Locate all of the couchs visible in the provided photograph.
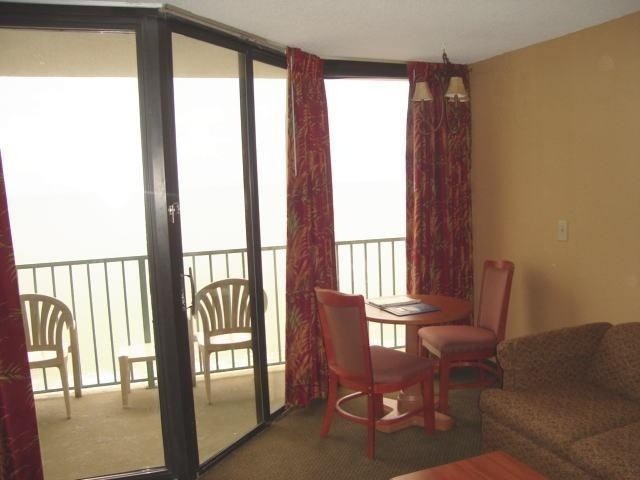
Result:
[476,321,640,480]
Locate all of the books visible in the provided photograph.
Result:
[364,295,441,317]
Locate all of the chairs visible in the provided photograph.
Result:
[118,277,269,410]
[15,293,81,421]
[313,285,435,461]
[419,260,514,414]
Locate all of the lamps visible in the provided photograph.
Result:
[409,48,472,136]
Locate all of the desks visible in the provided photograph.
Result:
[359,294,473,432]
